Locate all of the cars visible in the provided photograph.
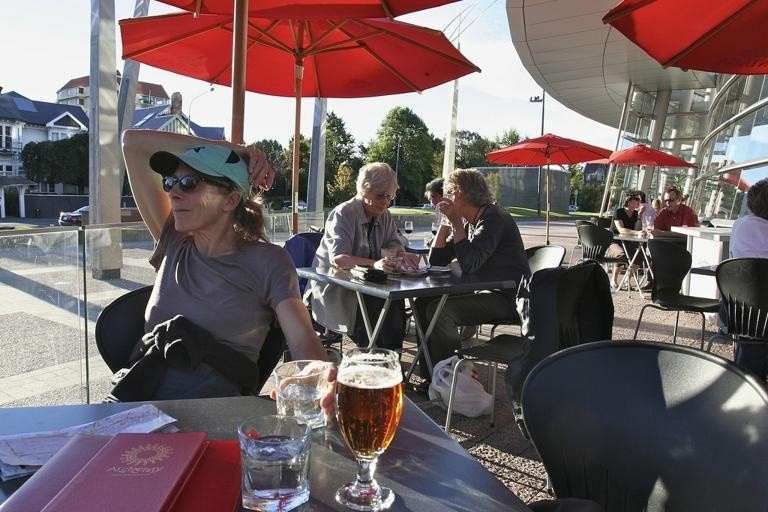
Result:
[58,205,90,226]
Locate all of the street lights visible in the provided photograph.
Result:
[187,87,215,136]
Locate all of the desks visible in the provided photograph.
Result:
[671,225,732,300]
[406,239,435,269]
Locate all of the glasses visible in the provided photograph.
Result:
[663,198,676,203]
[160,174,229,194]
[368,190,395,201]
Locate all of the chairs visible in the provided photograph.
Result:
[281,232,345,366]
[521,340,768,511]
[491,246,566,339]
[705,256,767,352]
[576,225,613,273]
[94,285,289,392]
[634,238,721,350]
[443,260,613,438]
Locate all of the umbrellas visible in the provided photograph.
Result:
[154,0,465,25]
[118,10,481,248]
[601,0,768,75]
[485,134,614,242]
[609,144,696,188]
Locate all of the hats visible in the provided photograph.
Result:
[149,143,252,197]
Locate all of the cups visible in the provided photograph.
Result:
[274,358,334,431]
[236,414,313,512]
[642,220,647,231]
[335,346,405,512]
[431,222,439,239]
[400,266,428,276]
[645,215,652,222]
[404,220,413,245]
[383,256,404,277]
[646,222,654,239]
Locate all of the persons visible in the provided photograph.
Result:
[94,129,329,405]
[424,177,464,247]
[728,177,768,259]
[300,163,419,366]
[608,196,647,292]
[635,191,657,239]
[640,187,700,290]
[651,197,664,212]
[413,168,532,392]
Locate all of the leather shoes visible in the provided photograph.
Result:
[610,280,622,290]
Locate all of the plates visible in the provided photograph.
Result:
[428,266,453,274]
